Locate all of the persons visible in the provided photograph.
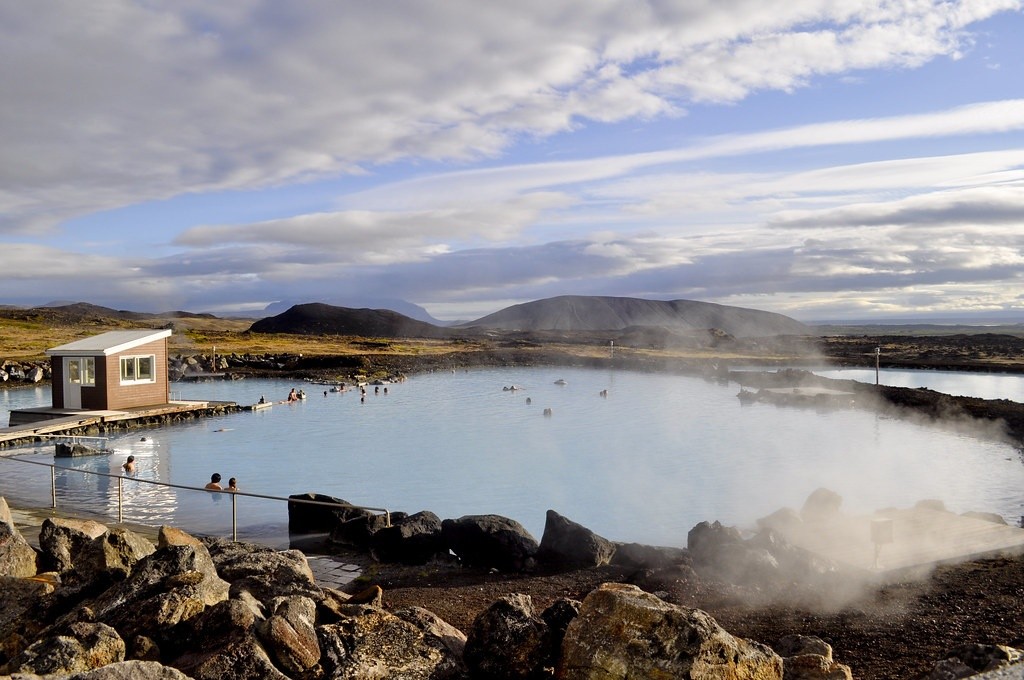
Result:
[123,456,135,472]
[259,371,408,403]
[206,473,240,492]
